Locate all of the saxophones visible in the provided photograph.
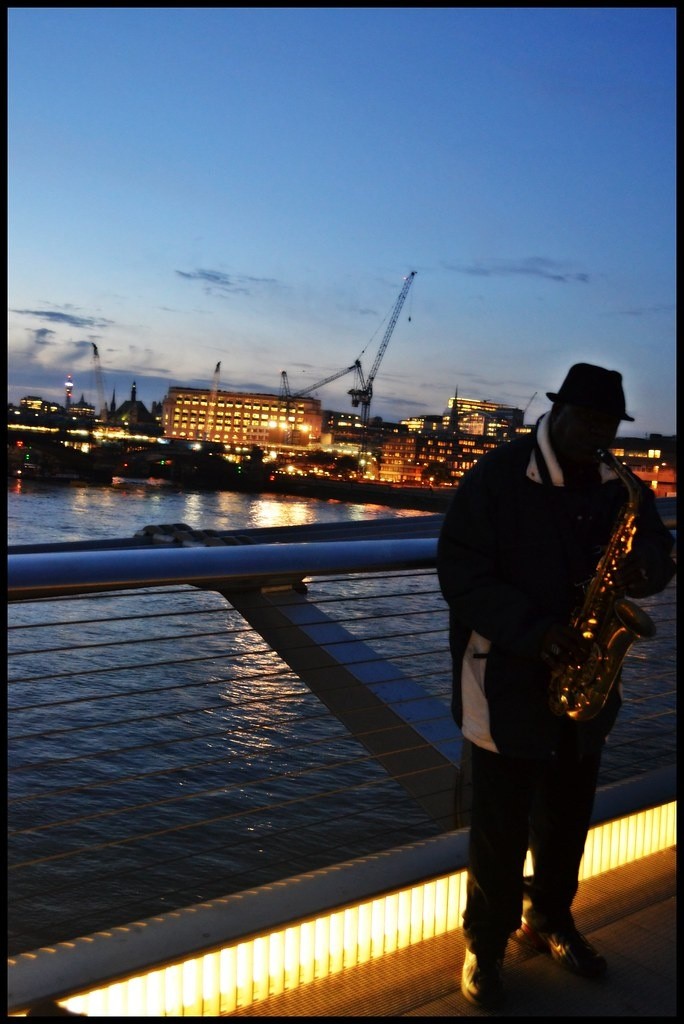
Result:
[548,448,657,721]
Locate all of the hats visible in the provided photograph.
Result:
[546,363,635,422]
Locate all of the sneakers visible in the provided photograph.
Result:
[461,937,506,1006]
[516,910,608,974]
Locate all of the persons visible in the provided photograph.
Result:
[433,363,678,1004]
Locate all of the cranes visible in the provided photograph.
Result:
[279,267,421,480]
[82,342,111,427]
